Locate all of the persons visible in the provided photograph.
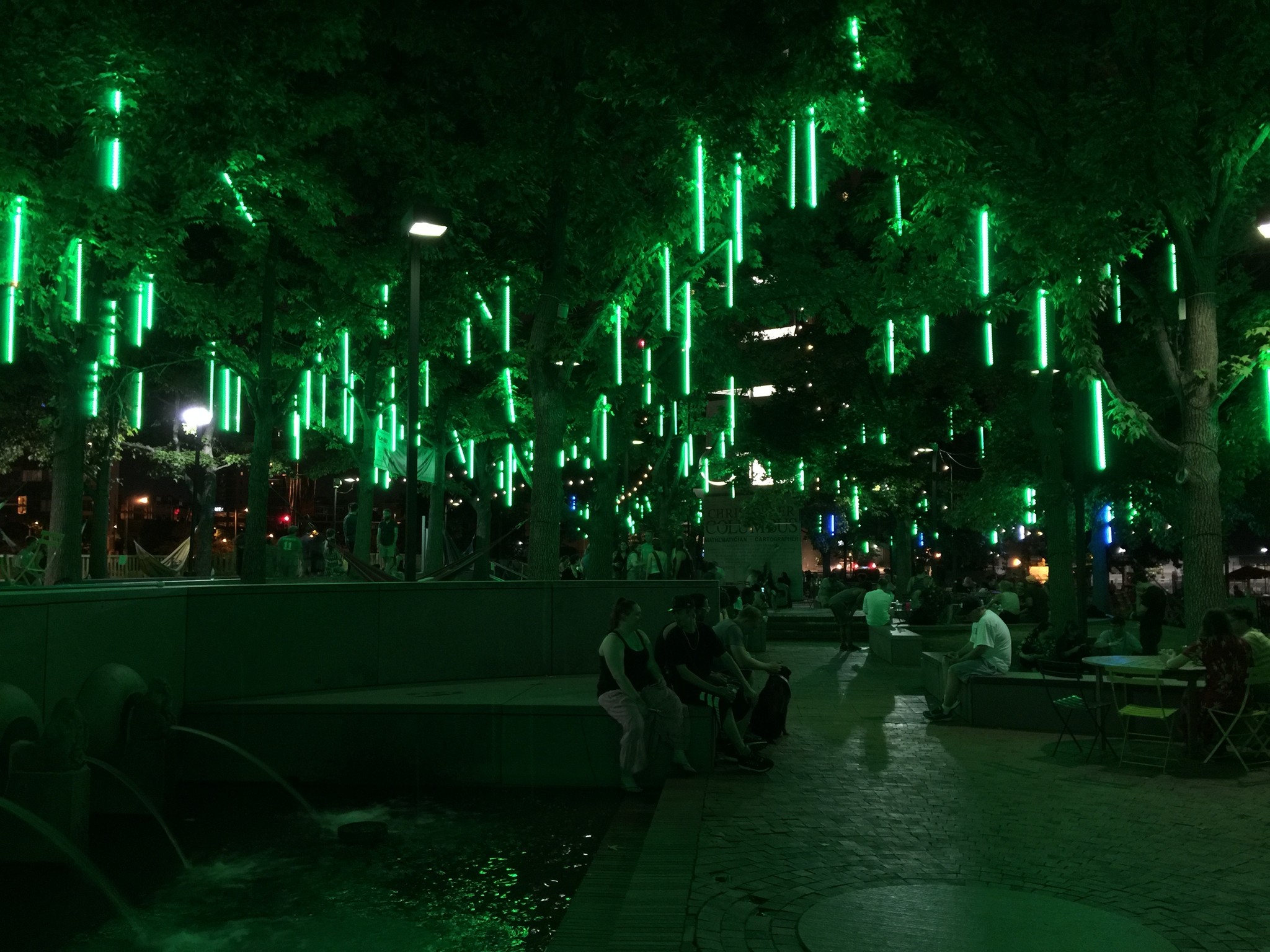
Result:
[907,555,1050,624]
[802,568,895,601]
[719,570,791,629]
[1166,608,1251,760]
[342,501,405,580]
[863,578,895,626]
[499,529,723,581]
[1236,606,1270,712]
[829,579,871,651]
[654,593,792,772]
[922,599,1011,721]
[1232,585,1244,598]
[211,524,344,577]
[598,595,697,794]
[1109,569,1184,655]
[1016,616,1143,675]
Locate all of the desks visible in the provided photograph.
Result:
[1081,655,1208,763]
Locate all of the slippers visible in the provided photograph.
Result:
[617,781,643,794]
[676,760,697,778]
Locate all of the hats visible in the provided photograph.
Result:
[878,577,890,585]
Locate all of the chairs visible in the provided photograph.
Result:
[1036,656,1119,762]
[1202,666,1270,771]
[803,584,819,611]
[890,602,907,624]
[1104,664,1183,772]
[11,530,65,587]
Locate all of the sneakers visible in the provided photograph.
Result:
[740,752,773,771]
[923,705,953,720]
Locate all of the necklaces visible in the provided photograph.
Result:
[681,625,700,651]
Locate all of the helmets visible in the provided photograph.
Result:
[668,595,695,612]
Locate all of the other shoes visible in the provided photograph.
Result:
[847,645,861,651]
[746,734,768,746]
[839,643,850,650]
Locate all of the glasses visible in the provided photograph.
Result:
[700,605,711,613]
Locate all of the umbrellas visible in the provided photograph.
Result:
[1225,565,1270,596]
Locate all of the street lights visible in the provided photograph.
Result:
[402,205,449,580]
